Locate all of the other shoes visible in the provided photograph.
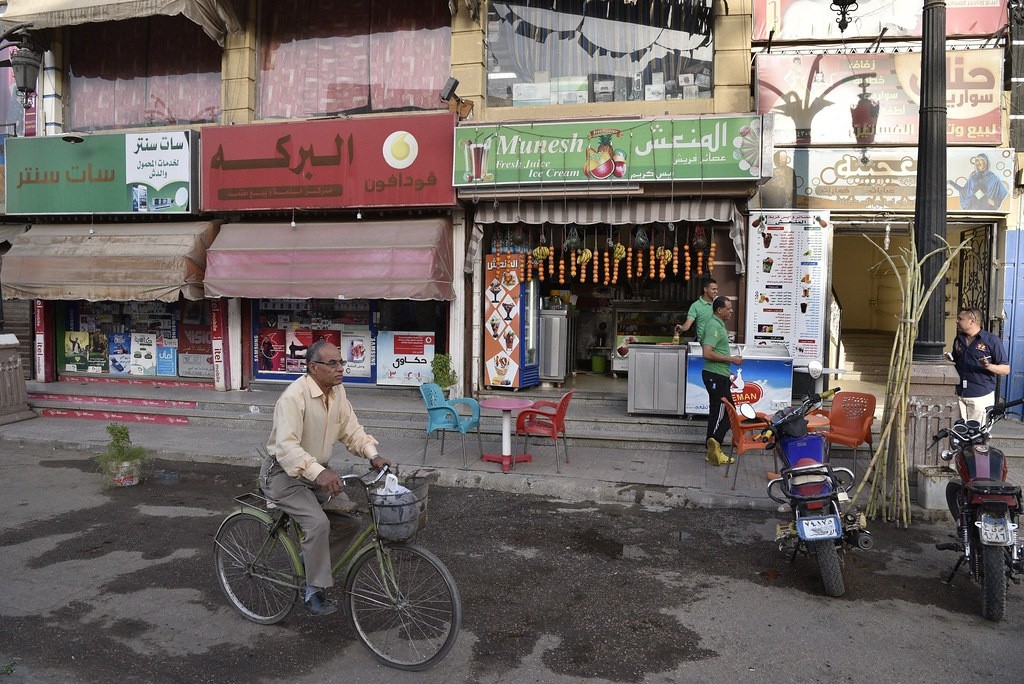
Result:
[706,438,720,466]
[704,448,735,465]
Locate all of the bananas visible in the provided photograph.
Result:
[577,255,581,264]
[663,249,672,265]
[533,246,549,260]
[581,249,592,262]
[613,244,626,260]
[656,246,661,259]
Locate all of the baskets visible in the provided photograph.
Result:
[369,482,430,540]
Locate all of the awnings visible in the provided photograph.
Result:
[203,217,457,301]
[0,218,223,303]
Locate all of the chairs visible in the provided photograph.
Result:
[511,387,576,473]
[808,392,877,481]
[722,396,779,489]
[420,382,482,468]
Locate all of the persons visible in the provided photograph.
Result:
[93,337,105,355]
[674,279,719,342]
[259,340,390,618]
[700,296,743,465]
[769,57,834,130]
[69,333,82,354]
[414,302,434,329]
[351,345,366,363]
[947,308,1010,474]
[261,336,275,370]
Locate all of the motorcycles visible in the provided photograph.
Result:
[740,360,874,596]
[926,398,1024,619]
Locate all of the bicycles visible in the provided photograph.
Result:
[213,463,462,672]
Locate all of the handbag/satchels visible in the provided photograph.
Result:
[374,474,422,541]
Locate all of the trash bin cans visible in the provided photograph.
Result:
[0,334,40,427]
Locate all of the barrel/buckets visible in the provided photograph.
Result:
[591,355,607,373]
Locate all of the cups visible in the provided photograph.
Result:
[465,144,490,179]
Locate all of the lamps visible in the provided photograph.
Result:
[356,210,361,219]
[89,215,93,234]
[9,27,40,108]
[290,209,295,227]
[884,222,891,250]
[830,0,858,32]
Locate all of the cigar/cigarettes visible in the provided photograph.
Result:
[981,356,991,360]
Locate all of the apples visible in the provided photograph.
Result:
[494,242,713,284]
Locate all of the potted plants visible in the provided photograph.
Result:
[97,422,151,490]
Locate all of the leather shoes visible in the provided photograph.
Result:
[302,590,338,615]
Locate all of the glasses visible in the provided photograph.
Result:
[312,358,347,369]
[960,307,977,321]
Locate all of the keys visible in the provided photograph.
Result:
[263,475,268,485]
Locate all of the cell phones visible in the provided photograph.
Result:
[132,185,148,212]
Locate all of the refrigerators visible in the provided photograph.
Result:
[685,341,793,420]
[485,255,540,387]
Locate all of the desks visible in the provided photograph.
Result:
[477,397,534,473]
[763,413,831,480]
